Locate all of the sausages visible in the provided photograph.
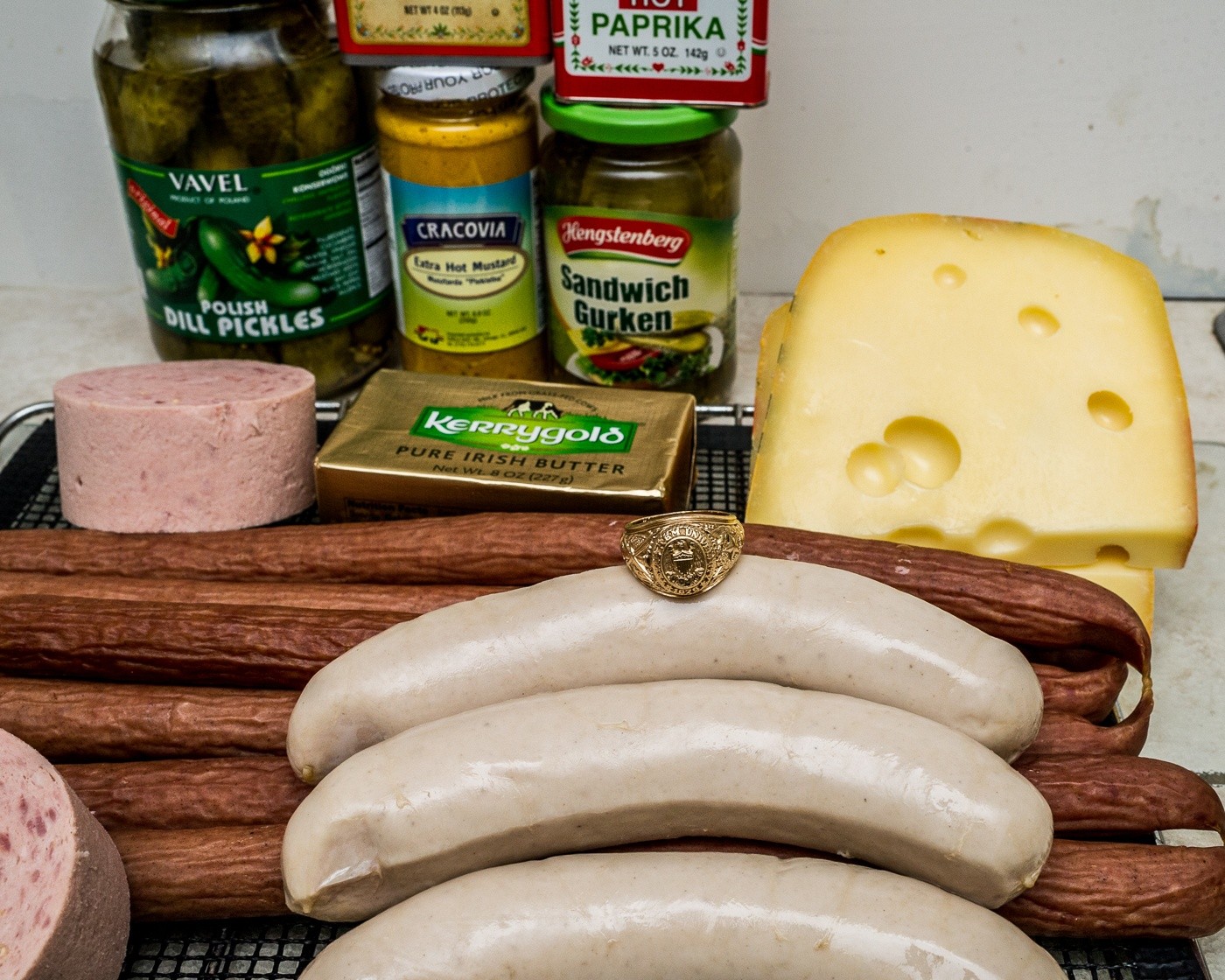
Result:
[0,516,1225,980]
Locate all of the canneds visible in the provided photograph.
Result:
[90,0,747,407]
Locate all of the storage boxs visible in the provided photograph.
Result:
[314,368,697,523]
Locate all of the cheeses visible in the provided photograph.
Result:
[745,210,1197,640]
